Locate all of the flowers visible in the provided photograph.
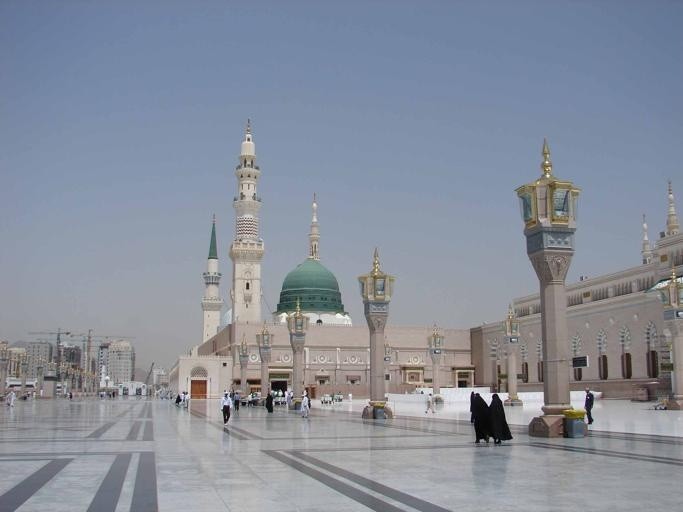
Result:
[372,402,385,409]
[562,408,586,421]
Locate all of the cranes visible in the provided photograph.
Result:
[27,329,136,373]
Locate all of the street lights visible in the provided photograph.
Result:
[255,320,274,406]
[514,135,581,440]
[656,261,683,410]
[284,295,310,410]
[357,246,397,420]
[503,303,523,406]
[237,334,250,399]
[426,323,444,404]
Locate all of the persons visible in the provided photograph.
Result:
[220,393,232,425]
[248,393,253,407]
[233,392,240,411]
[488,394,513,444]
[425,392,436,414]
[300,393,310,418]
[585,387,595,424]
[469,391,478,424]
[264,388,294,416]
[184,391,189,407]
[472,395,492,443]
